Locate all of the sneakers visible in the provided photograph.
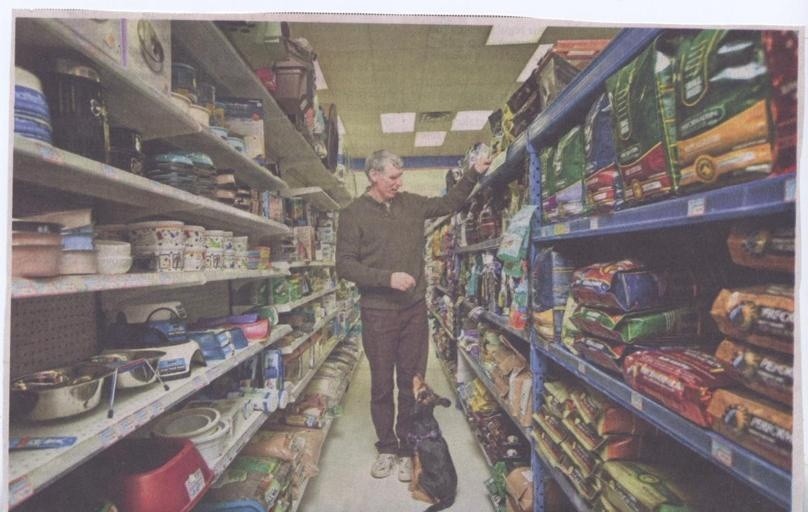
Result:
[372,454,398,478]
[399,456,414,481]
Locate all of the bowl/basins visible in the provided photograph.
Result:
[149,405,232,461]
[142,147,217,200]
[215,169,251,211]
[15,66,55,148]
[11,347,166,422]
[11,217,271,278]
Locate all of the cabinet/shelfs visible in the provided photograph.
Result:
[418,30,797,512]
[6,12,366,511]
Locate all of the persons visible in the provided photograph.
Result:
[335,149,492,482]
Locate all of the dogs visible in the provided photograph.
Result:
[406,372,458,512]
